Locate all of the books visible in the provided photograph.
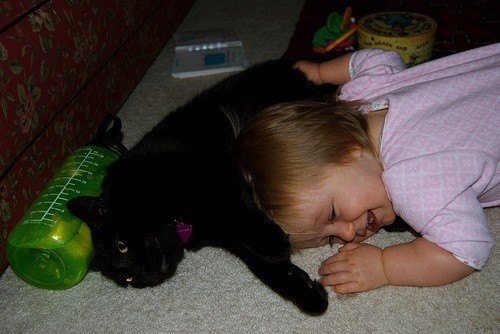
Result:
[170,29,247,79]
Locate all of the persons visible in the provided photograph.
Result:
[230,42,500,294]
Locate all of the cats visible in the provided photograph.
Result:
[66,55,341,317]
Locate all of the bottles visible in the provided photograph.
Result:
[6,113,128,290]
